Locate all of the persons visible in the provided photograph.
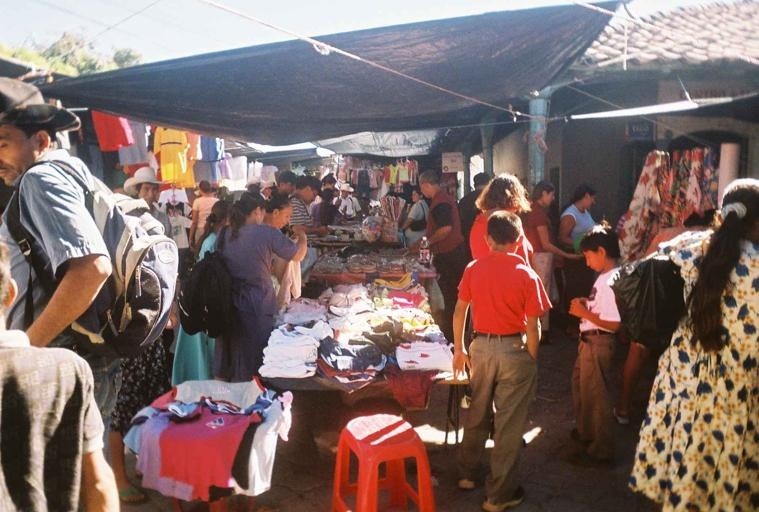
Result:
[108,335,170,505]
[468,172,535,267]
[275,169,297,199]
[0,234,121,511]
[612,208,718,426]
[557,184,598,342]
[261,194,294,328]
[0,76,125,467]
[171,199,233,385]
[523,179,585,345]
[187,178,221,250]
[567,231,624,461]
[333,183,362,220]
[402,187,430,247]
[451,209,554,512]
[123,166,172,240]
[457,172,492,265]
[310,188,348,226]
[211,190,309,383]
[410,169,465,325]
[627,176,759,512]
[288,175,329,238]
[322,172,337,191]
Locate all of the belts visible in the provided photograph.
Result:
[576,329,609,344]
[471,330,522,338]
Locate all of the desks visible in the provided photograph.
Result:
[261,367,469,452]
[311,270,440,300]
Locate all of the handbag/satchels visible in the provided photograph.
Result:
[410,203,426,232]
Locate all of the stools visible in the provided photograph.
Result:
[330,415,436,512]
[172,491,253,512]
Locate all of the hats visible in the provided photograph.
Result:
[123,166,164,195]
[0,76,81,138]
[338,183,354,192]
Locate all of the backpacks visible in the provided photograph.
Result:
[7,157,179,359]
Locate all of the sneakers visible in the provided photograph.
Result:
[482,486,524,511]
[458,475,476,489]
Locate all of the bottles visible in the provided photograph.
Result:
[418,236,431,262]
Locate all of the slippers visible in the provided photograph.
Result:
[612,406,630,424]
[118,486,146,502]
